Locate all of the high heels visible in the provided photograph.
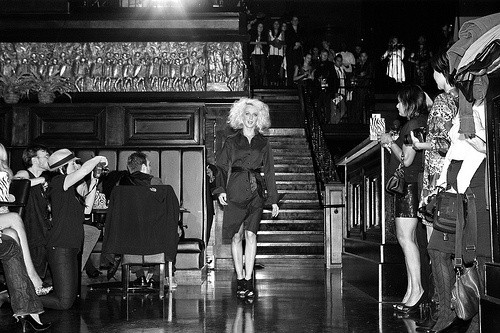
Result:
[391,303,422,311]
[424,314,458,333]
[19,313,52,333]
[416,313,437,333]
[396,289,427,316]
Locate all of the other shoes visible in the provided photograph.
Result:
[237,279,245,296]
[86,267,102,278]
[35,285,53,297]
[148,278,160,288]
[243,279,255,297]
[134,275,148,288]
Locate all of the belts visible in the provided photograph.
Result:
[231,166,261,174]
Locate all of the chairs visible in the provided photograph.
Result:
[1,179,31,218]
[109,184,175,300]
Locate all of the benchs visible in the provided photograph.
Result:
[69,145,206,269]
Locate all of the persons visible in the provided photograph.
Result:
[212,96,280,305]
[353,52,376,125]
[378,84,429,315]
[0,43,246,92]
[410,51,461,328]
[0,144,53,295]
[331,53,350,124]
[293,54,316,125]
[249,22,270,89]
[313,49,338,124]
[426,66,493,333]
[408,35,434,89]
[36,149,109,333]
[267,21,284,89]
[305,39,372,94]
[14,144,57,287]
[115,152,171,288]
[0,236,53,333]
[284,17,305,89]
[381,38,406,84]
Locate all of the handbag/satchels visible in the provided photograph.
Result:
[386,152,406,196]
[452,262,481,322]
[433,186,466,234]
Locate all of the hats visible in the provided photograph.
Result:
[48,148,79,171]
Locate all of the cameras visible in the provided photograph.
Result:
[93,162,106,178]
[404,127,426,144]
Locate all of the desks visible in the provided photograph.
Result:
[87,208,191,292]
